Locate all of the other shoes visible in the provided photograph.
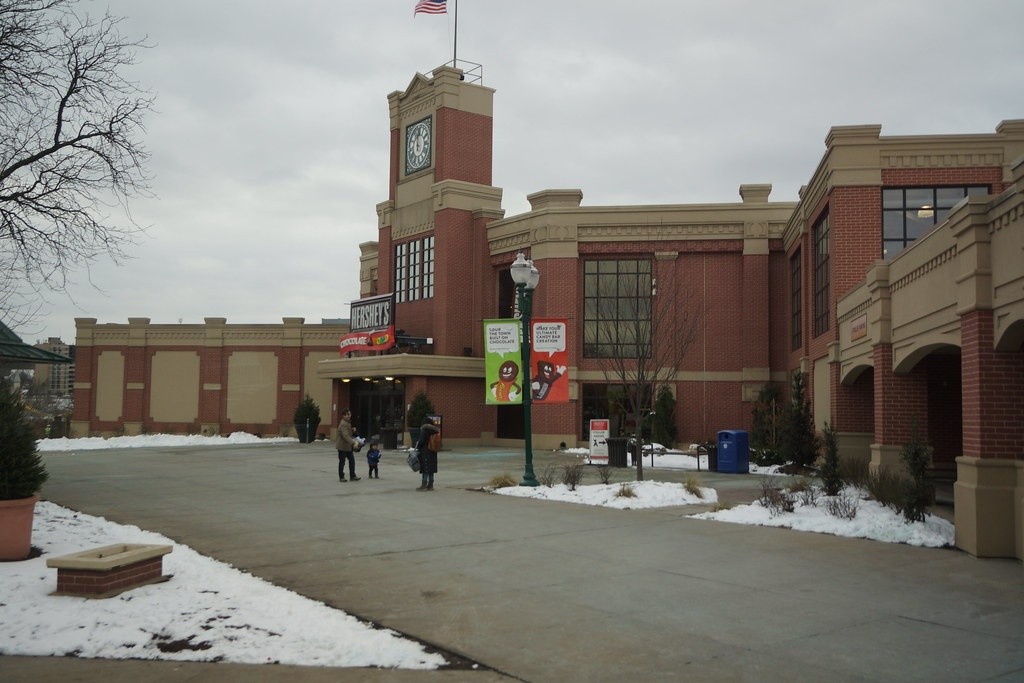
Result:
[341,478,347,482]
[369,476,373,478]
[350,476,361,481]
[375,476,379,479]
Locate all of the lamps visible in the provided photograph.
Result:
[918,205,934,217]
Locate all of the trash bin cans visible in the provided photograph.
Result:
[605,437,629,468]
[717,430,749,474]
[705,444,718,472]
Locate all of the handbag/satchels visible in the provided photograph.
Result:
[407,441,419,472]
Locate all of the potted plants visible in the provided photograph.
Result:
[405,391,433,447]
[0,376,52,561]
[293,394,322,443]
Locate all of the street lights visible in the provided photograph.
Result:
[510,250,541,487]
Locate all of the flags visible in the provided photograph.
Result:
[413,0,447,17]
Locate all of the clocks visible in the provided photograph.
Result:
[406,122,430,169]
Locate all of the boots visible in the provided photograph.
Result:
[428,480,434,489]
[416,481,428,491]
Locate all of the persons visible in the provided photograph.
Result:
[366,442,382,479]
[628,434,638,465]
[335,409,361,482]
[416,418,440,490]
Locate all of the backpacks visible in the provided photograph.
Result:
[428,428,442,452]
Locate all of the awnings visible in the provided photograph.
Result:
[0,322,73,366]
